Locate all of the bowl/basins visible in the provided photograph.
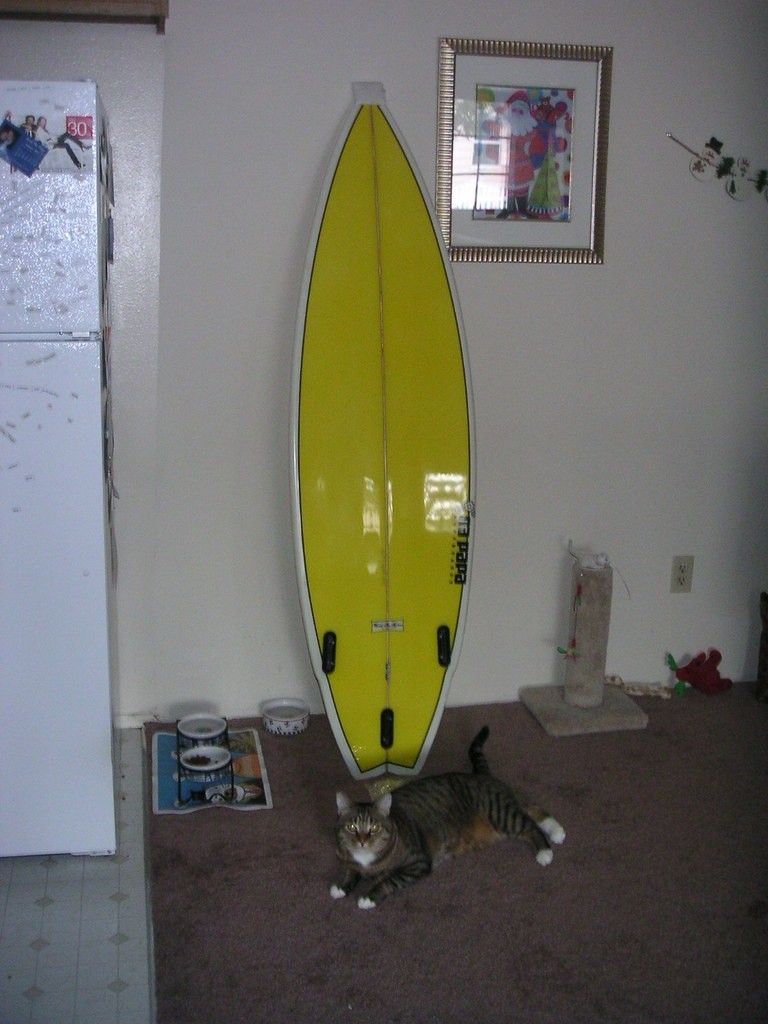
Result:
[178,714,226,749]
[263,698,309,734]
[179,747,231,783]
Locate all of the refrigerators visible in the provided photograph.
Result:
[0,80,119,857]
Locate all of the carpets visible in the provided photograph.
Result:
[143,682,768,1024]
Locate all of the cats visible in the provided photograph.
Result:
[329,726,565,910]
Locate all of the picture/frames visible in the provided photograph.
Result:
[435,36,613,264]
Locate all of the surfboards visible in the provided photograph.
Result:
[289,81,478,783]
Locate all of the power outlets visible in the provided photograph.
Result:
[670,556,694,593]
